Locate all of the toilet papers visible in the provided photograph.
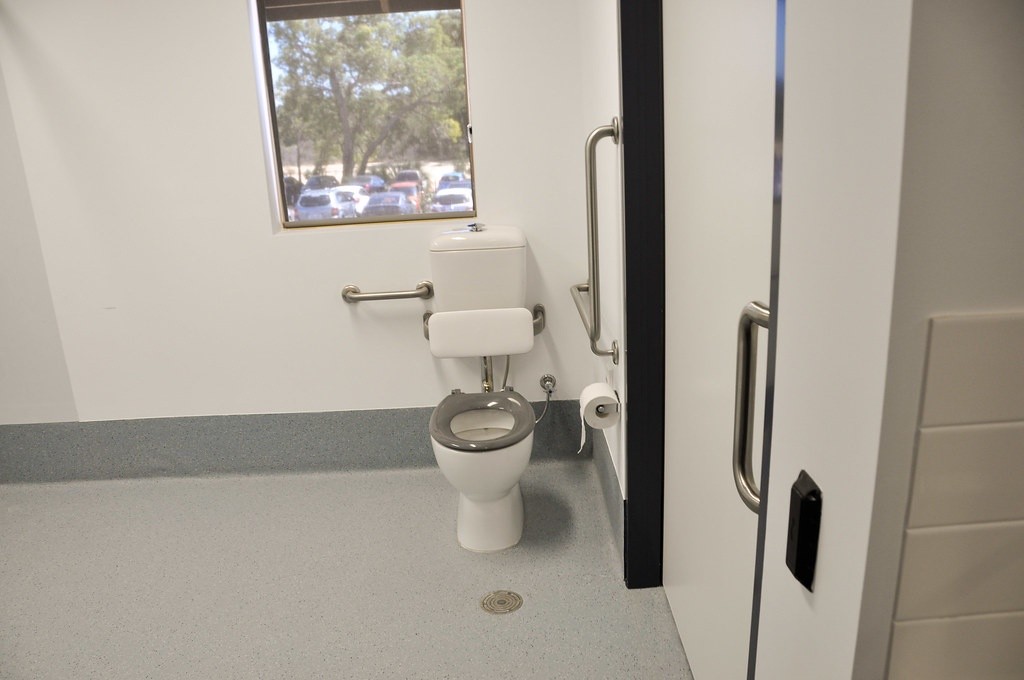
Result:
[576,384,620,453]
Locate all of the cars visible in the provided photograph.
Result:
[300,176,338,195]
[352,175,384,190]
[449,180,472,188]
[390,182,421,208]
[397,170,421,187]
[433,189,473,211]
[439,172,464,184]
[295,191,342,220]
[331,186,370,216]
[284,176,304,203]
[362,193,415,215]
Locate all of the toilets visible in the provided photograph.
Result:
[427,223,535,552]
